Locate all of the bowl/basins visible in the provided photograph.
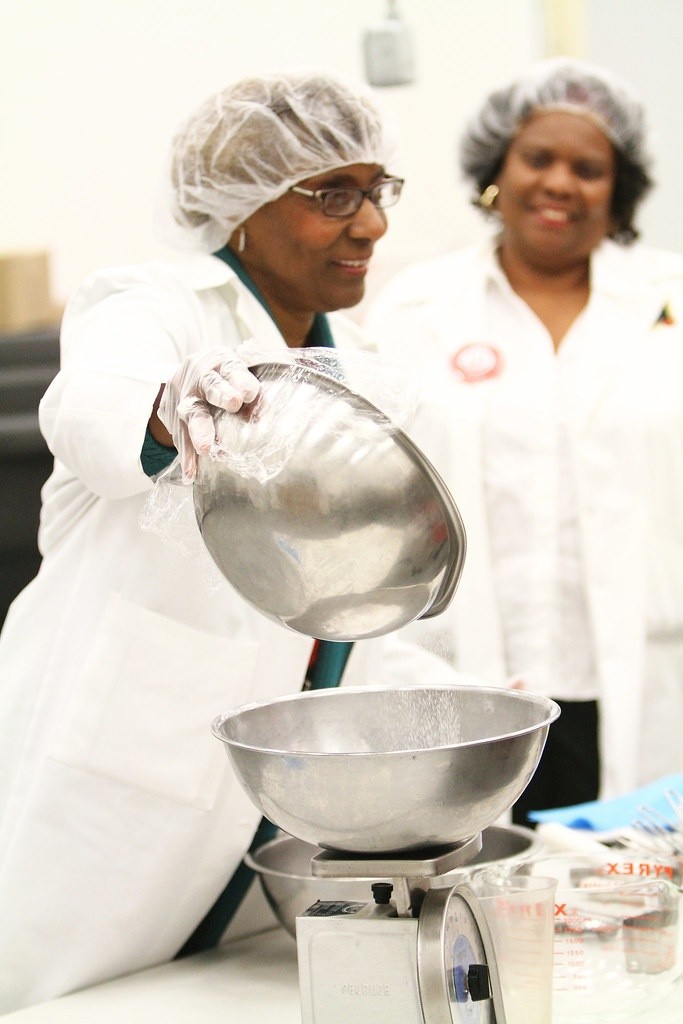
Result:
[195,365,467,642]
[212,682,560,853]
[244,823,542,938]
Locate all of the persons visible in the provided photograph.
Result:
[0,72,387,1012]
[338,56,683,829]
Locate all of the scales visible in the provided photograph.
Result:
[291,830,506,1024]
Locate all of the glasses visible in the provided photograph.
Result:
[290,174,404,216]
